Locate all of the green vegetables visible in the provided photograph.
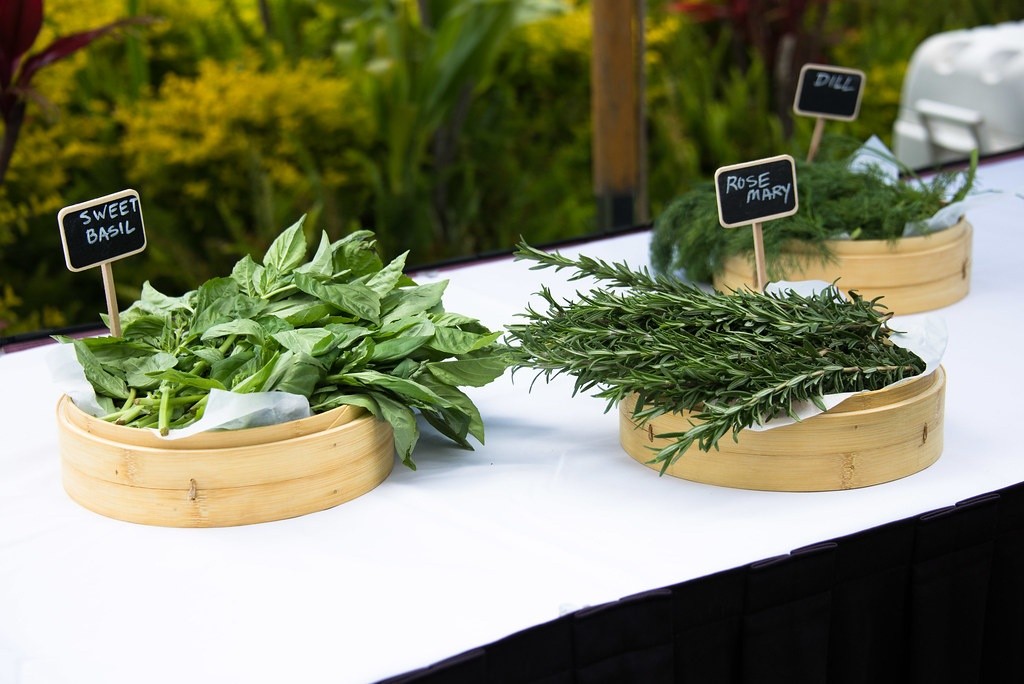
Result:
[493,234,927,477]
[647,133,978,281]
[50,212,528,471]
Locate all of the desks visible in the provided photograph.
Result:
[0,158,1024,684]
